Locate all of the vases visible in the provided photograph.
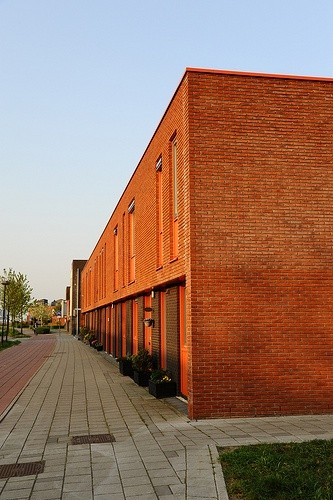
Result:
[148,381,178,398]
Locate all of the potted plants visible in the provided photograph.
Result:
[116,349,153,387]
[79,326,104,351]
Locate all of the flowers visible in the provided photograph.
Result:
[152,370,175,382]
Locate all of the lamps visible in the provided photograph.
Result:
[143,305,155,327]
